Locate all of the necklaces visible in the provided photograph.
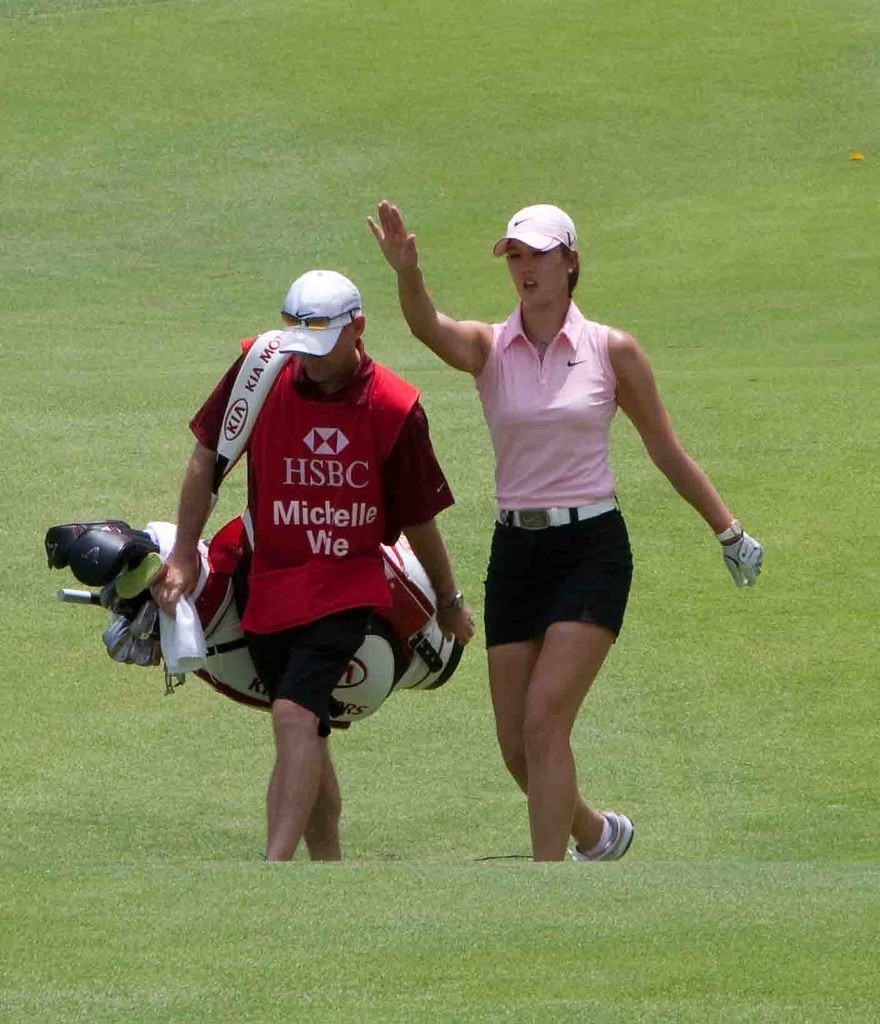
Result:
[525,330,557,350]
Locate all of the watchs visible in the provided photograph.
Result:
[716,519,744,542]
[436,591,464,616]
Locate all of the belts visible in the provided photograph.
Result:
[494,498,616,531]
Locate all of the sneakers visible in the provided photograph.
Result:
[567,809,634,861]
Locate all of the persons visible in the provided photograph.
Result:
[149,270,477,863]
[365,198,763,860]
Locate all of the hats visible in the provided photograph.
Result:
[492,204,577,259]
[279,270,361,358]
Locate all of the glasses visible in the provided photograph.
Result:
[280,306,362,331]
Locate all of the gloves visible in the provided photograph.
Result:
[721,530,765,588]
